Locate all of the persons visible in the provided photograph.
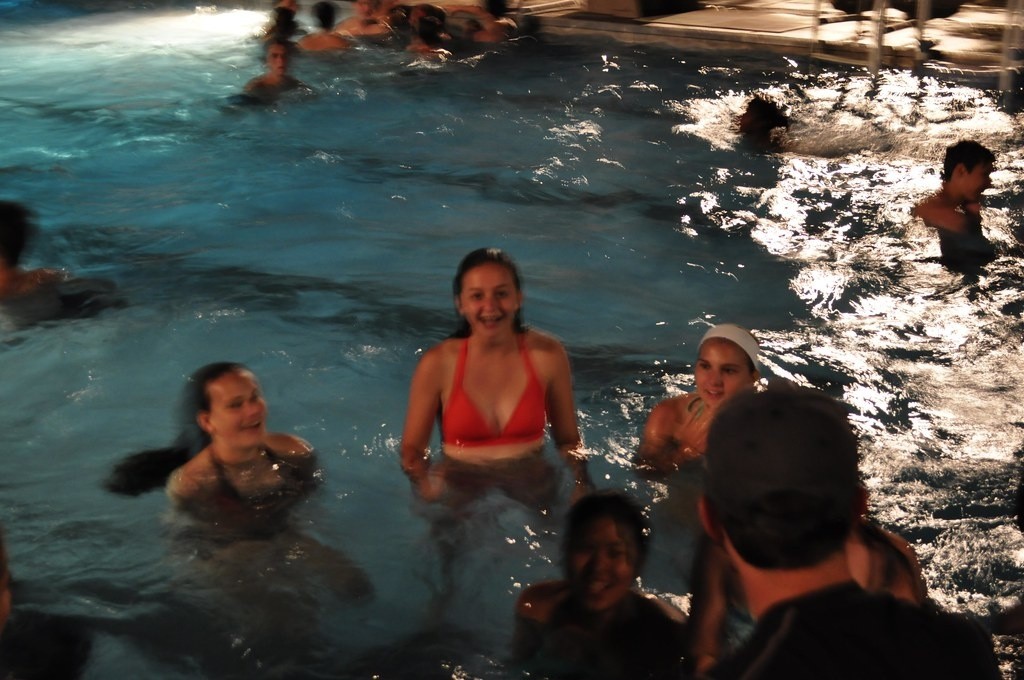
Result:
[509,325,1006,680]
[239,0,522,107]
[98,361,377,635]
[0,197,67,308]
[909,139,998,237]
[741,92,791,154]
[399,246,597,598]
